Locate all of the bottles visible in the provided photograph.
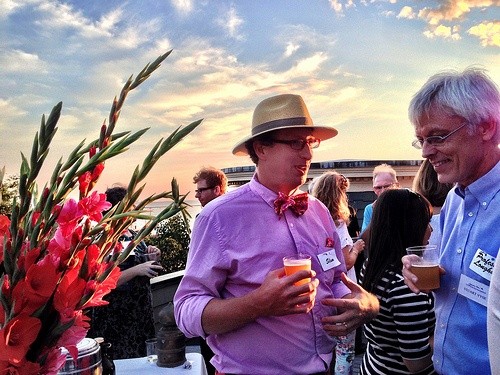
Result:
[94,337,115,375]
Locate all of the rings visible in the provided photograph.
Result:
[344,324,349,330]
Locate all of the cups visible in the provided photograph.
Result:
[145,338,158,364]
[283,254,312,296]
[405,245,440,291]
[144,253,156,262]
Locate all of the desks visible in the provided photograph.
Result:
[113,353,209,375]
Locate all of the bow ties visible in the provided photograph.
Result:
[274,192,309,220]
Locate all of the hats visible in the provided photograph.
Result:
[233,94,338,156]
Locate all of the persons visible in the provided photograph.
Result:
[308,172,366,375]
[193,168,228,207]
[89,186,163,375]
[358,189,437,375]
[346,206,365,277]
[401,64,500,375]
[173,94,380,375]
[361,164,399,235]
[413,159,455,262]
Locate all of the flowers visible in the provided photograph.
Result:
[0,49,203,375]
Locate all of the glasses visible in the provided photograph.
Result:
[269,138,320,150]
[412,122,468,148]
[195,186,214,194]
